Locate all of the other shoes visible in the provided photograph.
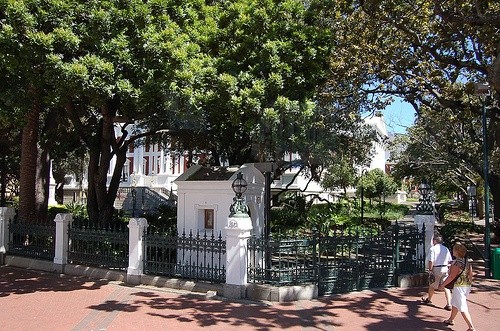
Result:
[444,305,451,311]
[422,298,432,303]
[444,320,455,326]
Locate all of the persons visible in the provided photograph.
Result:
[438,244,476,331]
[420,234,453,311]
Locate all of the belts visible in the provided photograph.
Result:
[433,265,447,267]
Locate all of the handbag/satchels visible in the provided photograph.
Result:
[444,280,455,290]
